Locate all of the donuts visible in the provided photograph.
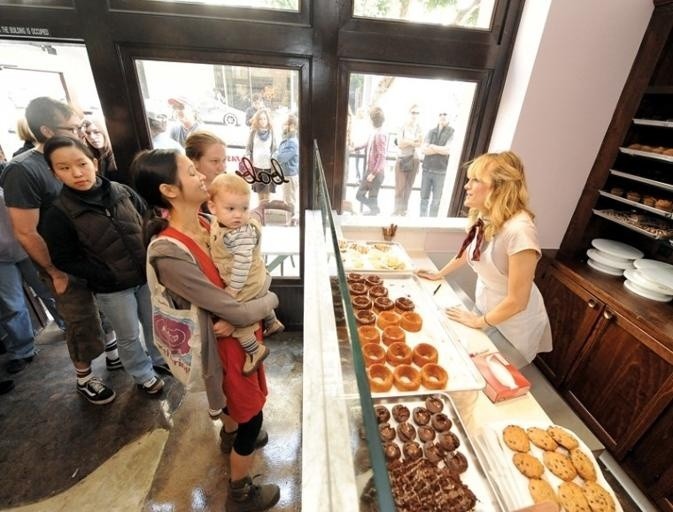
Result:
[355,398,477,470]
[330,272,448,391]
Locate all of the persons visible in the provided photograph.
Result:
[390,103,423,217]
[354,105,385,216]
[204,173,286,374]
[128,147,285,510]
[1,98,174,404]
[419,108,455,218]
[140,86,297,219]
[412,150,555,367]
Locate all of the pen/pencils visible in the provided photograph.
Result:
[382,223,397,241]
[433,284,442,296]
[470,349,490,357]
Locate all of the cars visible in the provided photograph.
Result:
[195,93,247,128]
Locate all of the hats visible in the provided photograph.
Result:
[167,94,195,108]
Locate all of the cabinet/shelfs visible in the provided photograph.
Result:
[608,371,673,511]
[528,245,672,458]
[550,1,671,283]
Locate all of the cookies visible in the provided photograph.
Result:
[504,426,615,512]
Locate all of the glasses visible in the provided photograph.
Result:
[171,104,186,112]
[52,120,89,137]
[439,113,447,117]
[412,111,420,115]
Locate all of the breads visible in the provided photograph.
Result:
[629,143,673,155]
[359,457,477,512]
[611,188,673,213]
[601,208,672,236]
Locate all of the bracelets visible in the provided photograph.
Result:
[483,314,494,328]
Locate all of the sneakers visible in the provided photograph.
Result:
[137,376,166,396]
[263,319,285,337]
[220,426,268,454]
[76,375,116,405]
[242,344,270,377]
[229,475,280,511]
[106,356,123,370]
[7,355,34,373]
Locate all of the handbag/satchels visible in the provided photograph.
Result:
[150,284,206,393]
[398,155,414,171]
